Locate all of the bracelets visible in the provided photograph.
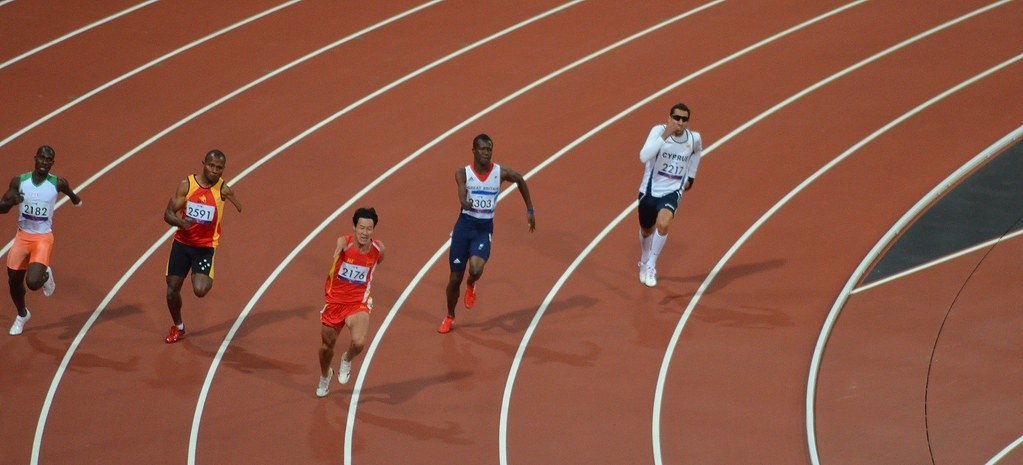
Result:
[527,208,534,213]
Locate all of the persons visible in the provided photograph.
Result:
[0,145,82,335]
[314,206,383,398]
[162,151,242,344]
[637,103,702,287]
[438,134,536,335]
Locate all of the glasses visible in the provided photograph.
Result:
[670,115,688,121]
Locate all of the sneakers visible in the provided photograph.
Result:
[338,351,352,384]
[464,287,475,308]
[645,267,656,286]
[165,325,185,343]
[638,261,647,284]
[437,314,454,333]
[9,308,31,335]
[316,367,333,396]
[42,266,56,297]
